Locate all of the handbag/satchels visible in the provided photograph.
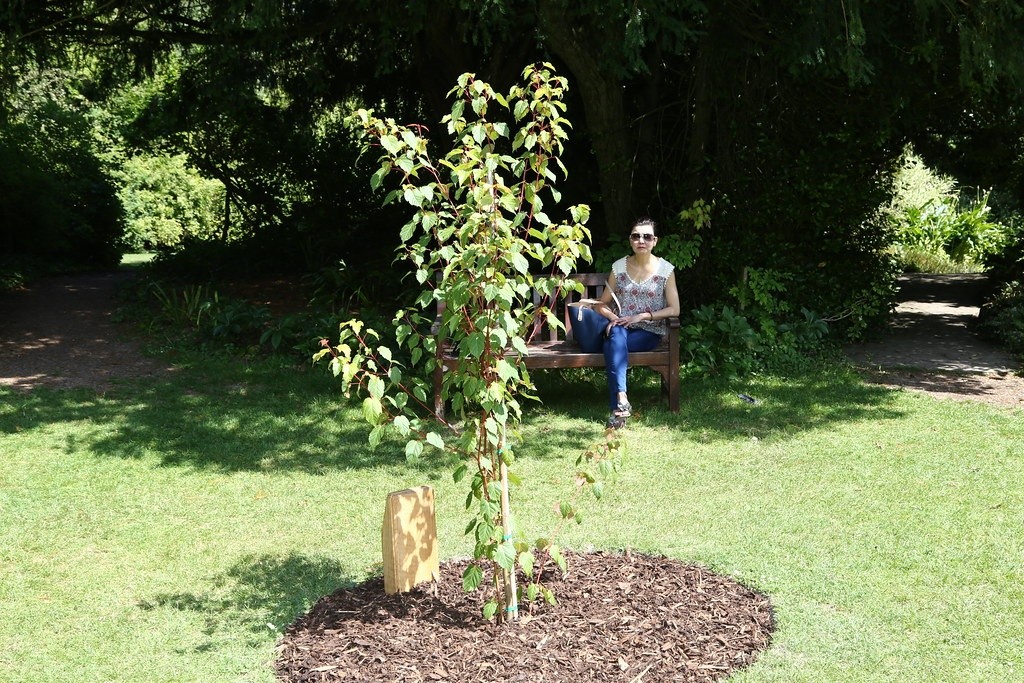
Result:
[567,299,612,353]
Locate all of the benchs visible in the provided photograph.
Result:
[432,273,681,417]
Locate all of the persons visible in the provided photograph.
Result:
[594,218,680,428]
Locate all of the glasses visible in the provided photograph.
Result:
[629,232,656,242]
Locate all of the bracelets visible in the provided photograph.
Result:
[647,311,653,321]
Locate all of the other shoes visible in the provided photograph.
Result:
[614,401,632,418]
[606,417,625,431]
